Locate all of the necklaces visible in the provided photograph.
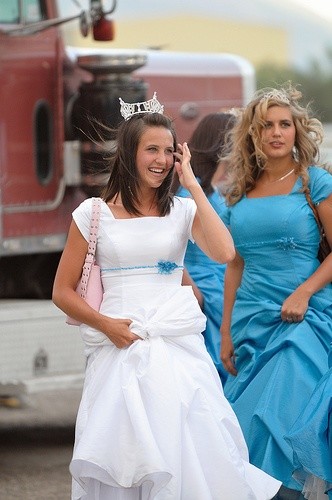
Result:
[274,168,295,184]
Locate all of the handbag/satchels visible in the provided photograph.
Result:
[300,161,332,264]
[66,263,103,326]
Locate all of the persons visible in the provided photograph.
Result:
[220,81,332,500]
[170,110,239,388]
[50,111,285,500]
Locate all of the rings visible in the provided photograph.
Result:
[286,318,293,321]
